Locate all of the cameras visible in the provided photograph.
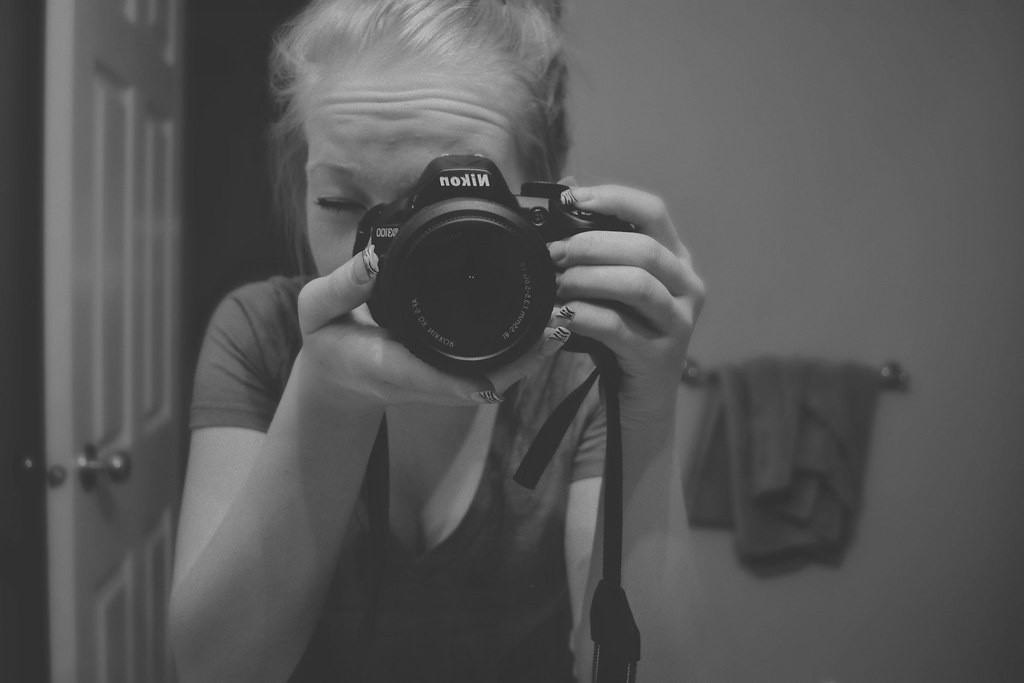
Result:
[352,156,640,372]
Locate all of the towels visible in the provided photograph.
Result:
[679,356,876,575]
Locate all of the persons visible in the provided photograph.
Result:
[162,1,707,683]
